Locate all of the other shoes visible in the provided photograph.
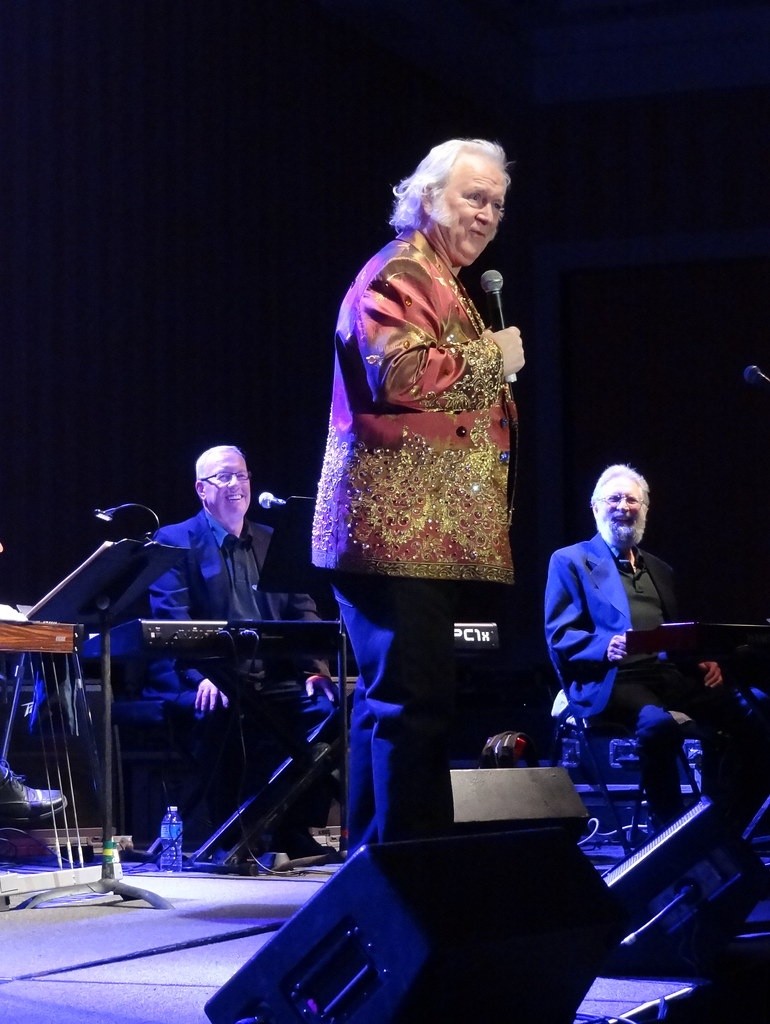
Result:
[287,831,335,859]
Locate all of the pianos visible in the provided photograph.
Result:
[80,619,350,874]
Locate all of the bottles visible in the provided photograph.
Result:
[160,805,183,874]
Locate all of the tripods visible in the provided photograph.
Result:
[12,547,190,914]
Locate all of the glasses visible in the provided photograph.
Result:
[202,470,250,483]
[597,494,648,508]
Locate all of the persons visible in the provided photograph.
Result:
[538,461,770,836]
[305,138,525,852]
[144,442,347,850]
[0,765,68,824]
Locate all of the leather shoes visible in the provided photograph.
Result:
[1,769,67,822]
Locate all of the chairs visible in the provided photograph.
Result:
[159,642,285,824]
[537,635,701,859]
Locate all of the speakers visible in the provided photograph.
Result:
[449,768,591,846]
[596,790,770,980]
[204,824,631,1024]
[5,676,107,832]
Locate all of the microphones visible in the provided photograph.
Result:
[744,366,770,389]
[259,492,287,509]
[481,270,517,382]
